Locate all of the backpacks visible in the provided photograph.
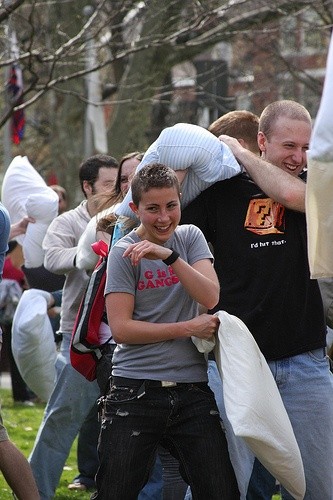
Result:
[69,240,113,382]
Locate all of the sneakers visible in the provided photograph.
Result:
[68,482,87,489]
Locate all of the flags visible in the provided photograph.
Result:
[83,34,110,156]
[7,32,25,146]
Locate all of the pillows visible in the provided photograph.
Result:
[2,155,60,268]
[11,288,59,402]
[191,311,307,500]
[115,122,242,225]
[77,202,123,270]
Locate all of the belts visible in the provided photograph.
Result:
[111,376,184,399]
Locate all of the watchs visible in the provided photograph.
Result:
[162,250,182,265]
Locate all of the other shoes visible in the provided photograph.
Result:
[14,402,35,407]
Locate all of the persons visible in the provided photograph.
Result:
[206,110,275,500]
[306,29,332,373]
[0,152,146,404]
[47,290,99,490]
[25,152,145,500]
[0,415,39,500]
[91,164,241,500]
[178,99,332,500]
